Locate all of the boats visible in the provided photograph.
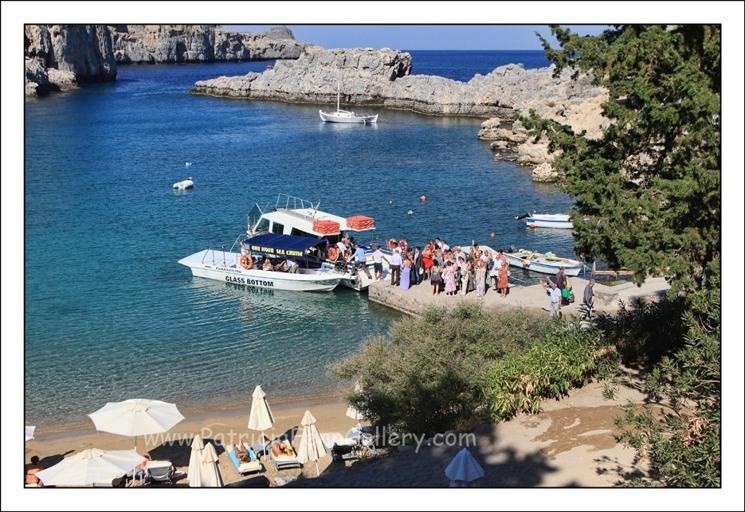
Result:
[501,249,585,277]
[176,190,408,294]
[459,244,510,279]
[172,176,194,191]
[515,212,591,229]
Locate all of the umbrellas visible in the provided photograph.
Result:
[186,434,225,488]
[87,398,186,485]
[248,384,276,461]
[33,448,147,487]
[25,425,37,441]
[346,374,370,442]
[297,409,329,485]
[444,447,485,482]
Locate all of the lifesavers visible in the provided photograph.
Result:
[328,247,338,260]
[239,255,253,269]
[387,238,409,255]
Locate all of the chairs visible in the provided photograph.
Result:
[144,459,176,487]
[321,431,357,449]
[269,440,301,470]
[226,442,262,473]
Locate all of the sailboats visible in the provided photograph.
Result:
[318,80,379,125]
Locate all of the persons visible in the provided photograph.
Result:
[270,437,293,457]
[540,266,567,318]
[583,279,595,309]
[333,237,511,299]
[25,455,45,488]
[491,232,497,240]
[131,452,151,477]
[232,439,249,464]
[262,258,300,274]
[420,195,426,202]
